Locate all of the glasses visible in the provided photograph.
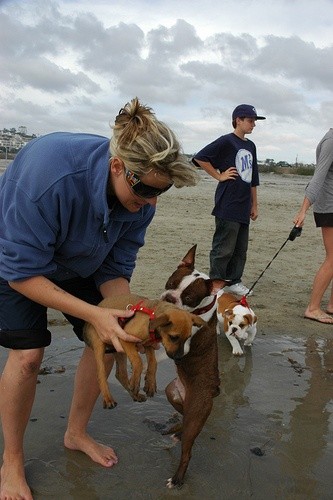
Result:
[124,167,174,199]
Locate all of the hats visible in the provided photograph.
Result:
[231,104,266,120]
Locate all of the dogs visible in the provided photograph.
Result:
[158,244,226,489]
[216,289,258,356]
[83,291,206,410]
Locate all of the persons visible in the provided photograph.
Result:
[193,104,266,296]
[293,129,333,324]
[1,98,199,500]
